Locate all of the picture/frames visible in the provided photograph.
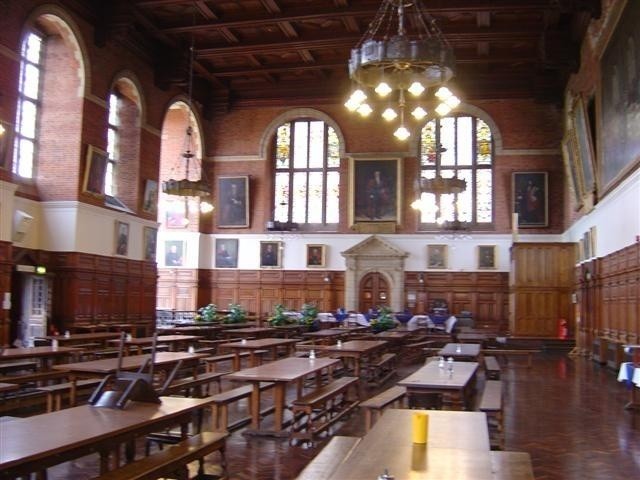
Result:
[477,245,497,270]
[81,143,162,265]
[508,167,553,230]
[306,243,325,268]
[217,173,252,231]
[163,238,186,268]
[346,151,406,231]
[215,238,238,268]
[574,225,598,267]
[552,1,640,209]
[163,195,190,231]
[260,241,283,269]
[427,244,448,270]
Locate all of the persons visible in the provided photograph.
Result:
[366,171,385,219]
[168,245,182,265]
[263,244,274,265]
[227,183,243,225]
[219,244,232,265]
[525,180,540,223]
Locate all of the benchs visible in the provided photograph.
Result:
[599,335,640,410]
[0,290,534,480]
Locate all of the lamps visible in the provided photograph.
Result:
[440,193,472,235]
[267,144,302,236]
[160,36,216,231]
[338,0,464,143]
[408,103,468,228]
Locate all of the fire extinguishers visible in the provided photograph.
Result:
[558,317,569,340]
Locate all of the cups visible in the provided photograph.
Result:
[412,412,428,444]
[411,442,428,473]
[456,344,462,352]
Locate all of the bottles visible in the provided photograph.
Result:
[186,344,197,359]
[334,339,342,354]
[432,353,453,373]
[240,335,247,349]
[125,332,132,344]
[51,337,60,353]
[306,348,316,366]
[376,469,396,480]
